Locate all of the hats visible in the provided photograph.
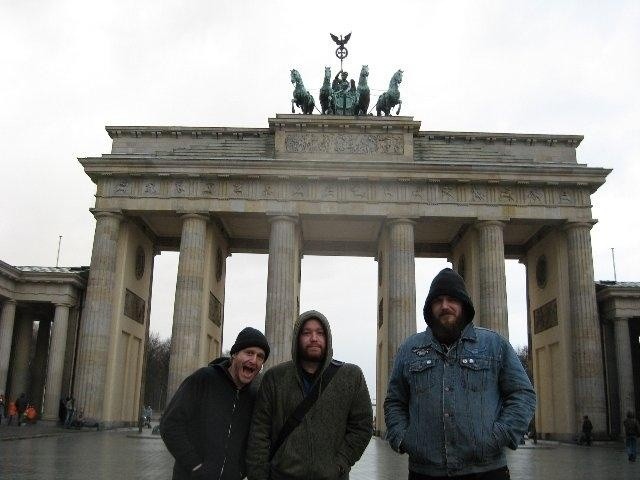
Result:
[230,328,270,363]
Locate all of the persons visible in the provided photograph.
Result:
[0,391,37,429]
[146,404,153,424]
[333,70,348,92]
[623,410,640,463]
[242,310,374,479]
[58,392,85,428]
[579,416,594,446]
[158,326,271,479]
[381,267,537,480]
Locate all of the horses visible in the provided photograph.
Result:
[376,69,405,117]
[288,68,315,115]
[357,64,371,114]
[319,65,333,115]
